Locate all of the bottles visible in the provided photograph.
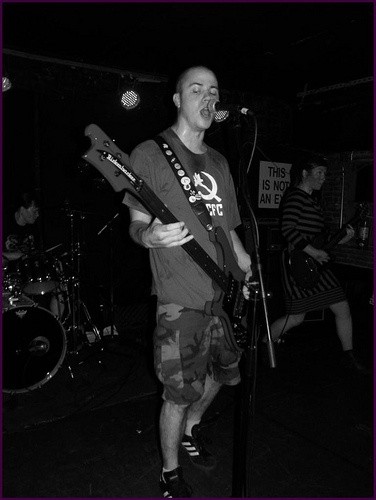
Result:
[359,217,369,248]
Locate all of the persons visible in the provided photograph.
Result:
[123,68,251,500]
[0,192,83,355]
[260,155,368,372]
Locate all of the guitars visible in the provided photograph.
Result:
[81,123,278,354]
[286,205,371,291]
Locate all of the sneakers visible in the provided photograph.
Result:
[159,465,191,498]
[181,423,217,470]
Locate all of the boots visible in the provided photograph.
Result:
[255,324,270,365]
[340,349,369,376]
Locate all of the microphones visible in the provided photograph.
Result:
[207,99,255,116]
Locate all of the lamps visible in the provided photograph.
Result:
[215,110,230,124]
[3,64,13,92]
[116,73,141,109]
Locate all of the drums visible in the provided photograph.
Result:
[2,293,69,395]
[14,249,63,296]
[0,255,22,294]
